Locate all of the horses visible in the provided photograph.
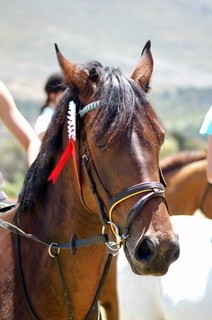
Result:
[0,40,180,320]
[159,151,211,219]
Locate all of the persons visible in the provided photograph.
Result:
[1,80,43,213]
[198,104,212,186]
[31,70,67,143]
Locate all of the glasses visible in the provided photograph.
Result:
[57,87,69,93]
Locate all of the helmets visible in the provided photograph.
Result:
[45,73,67,91]
